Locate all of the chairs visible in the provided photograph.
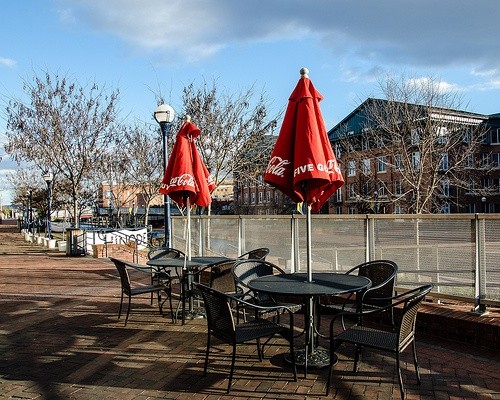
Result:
[109,246,435,400]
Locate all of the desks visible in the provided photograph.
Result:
[146,257,231,320]
[247,273,372,370]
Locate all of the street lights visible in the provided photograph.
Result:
[154,103,175,253]
[42,171,54,239]
